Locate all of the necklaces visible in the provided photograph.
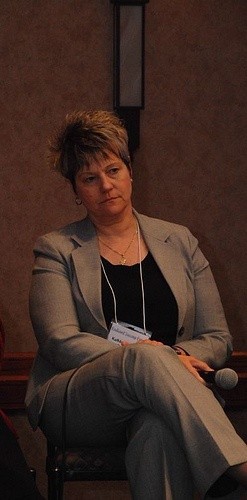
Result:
[96,223,138,266]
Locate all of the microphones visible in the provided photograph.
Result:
[196,369,239,388]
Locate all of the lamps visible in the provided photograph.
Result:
[110,0,150,162]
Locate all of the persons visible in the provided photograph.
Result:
[25,106,247,500]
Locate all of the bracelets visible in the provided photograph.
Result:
[171,346,182,356]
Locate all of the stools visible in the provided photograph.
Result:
[46,432,129,500]
